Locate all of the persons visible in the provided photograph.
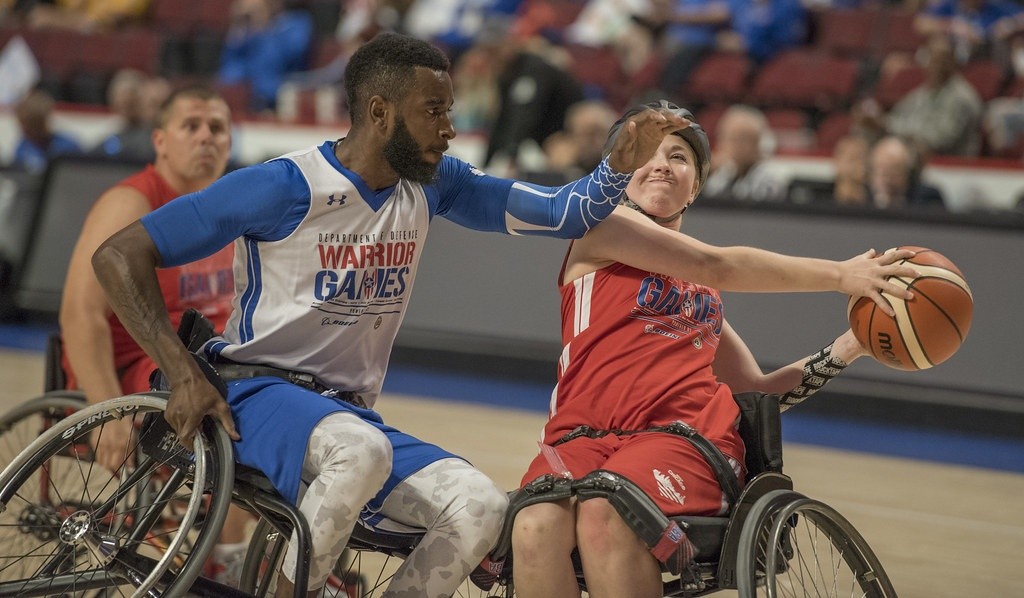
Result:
[0,1,1024,219]
[57,87,260,598]
[509,100,918,598]
[93,31,690,598]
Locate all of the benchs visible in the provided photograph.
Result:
[0,0,1024,221]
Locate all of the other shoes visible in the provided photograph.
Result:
[202,541,250,589]
[320,572,366,598]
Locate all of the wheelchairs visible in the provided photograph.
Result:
[449,392,900,598]
[0,309,451,598]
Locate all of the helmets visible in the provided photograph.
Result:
[602,98,711,203]
